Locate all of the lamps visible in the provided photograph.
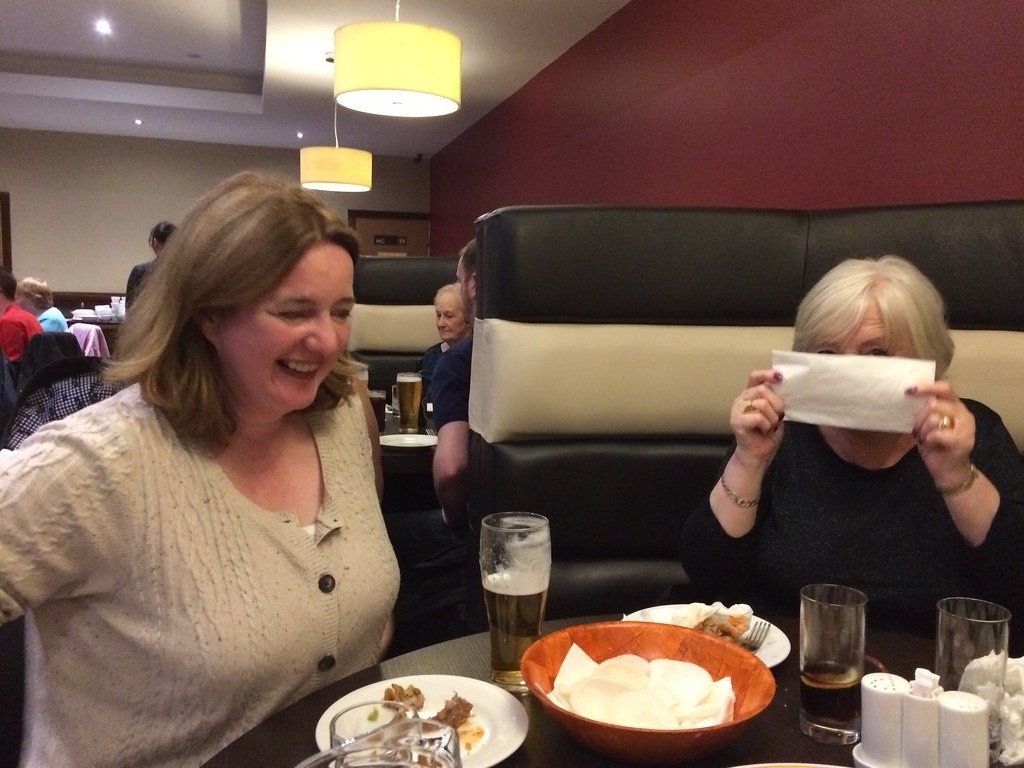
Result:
[331,0,465,121]
[299,50,375,194]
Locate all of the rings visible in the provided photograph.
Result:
[940,415,954,429]
[745,399,757,413]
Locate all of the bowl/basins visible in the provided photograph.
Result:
[97,315,115,322]
[81,316,97,323]
[521,621,776,760]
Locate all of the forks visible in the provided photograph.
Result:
[425,428,436,436]
[739,622,771,655]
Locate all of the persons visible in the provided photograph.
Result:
[0,266,44,393]
[677,251,1024,646]
[0,171,400,768]
[413,280,473,440]
[13,275,68,333]
[431,236,479,556]
[124,221,180,312]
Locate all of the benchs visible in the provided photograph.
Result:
[346,256,460,401]
[455,197,1024,632]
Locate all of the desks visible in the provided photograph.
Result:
[199,606,1024,768]
[67,320,123,355]
[381,432,439,475]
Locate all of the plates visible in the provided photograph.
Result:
[379,433,438,447]
[621,604,791,668]
[315,675,528,768]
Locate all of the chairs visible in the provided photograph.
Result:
[19,331,85,394]
[5,349,130,445]
[68,322,107,357]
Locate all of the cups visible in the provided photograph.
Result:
[799,584,868,744]
[391,385,400,418]
[396,373,422,433]
[369,389,387,435]
[329,700,464,768]
[935,597,1011,766]
[479,511,552,697]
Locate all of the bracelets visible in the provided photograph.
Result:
[721,477,761,508]
[939,462,979,496]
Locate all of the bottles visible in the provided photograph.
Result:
[937,691,989,768]
[904,682,945,768]
[860,673,911,768]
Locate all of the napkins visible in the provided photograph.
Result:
[769,350,937,433]
